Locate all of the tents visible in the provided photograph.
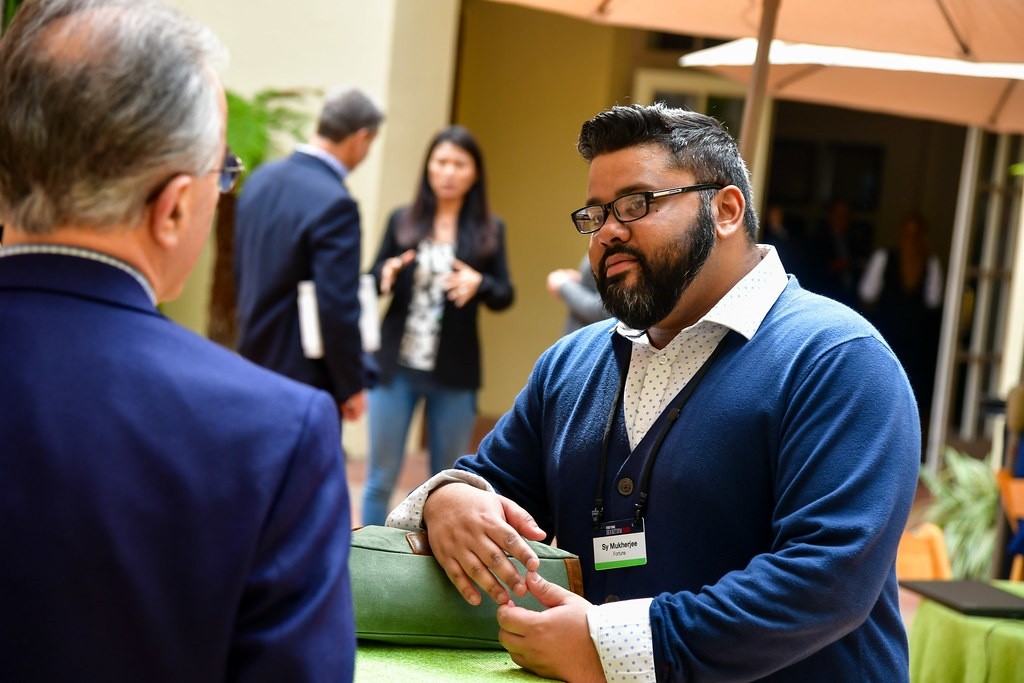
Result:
[489,0,1024,475]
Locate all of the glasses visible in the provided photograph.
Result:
[142,156,242,205]
[571,183,723,235]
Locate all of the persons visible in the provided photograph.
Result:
[360,127,516,528]
[0,0,357,683]
[547,254,612,336]
[810,201,861,278]
[385,104,921,683]
[233,88,384,423]
[860,214,943,372]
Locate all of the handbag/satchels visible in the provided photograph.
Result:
[350,521,582,650]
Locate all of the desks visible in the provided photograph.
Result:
[910,579,1024,683]
[349,639,564,683]
